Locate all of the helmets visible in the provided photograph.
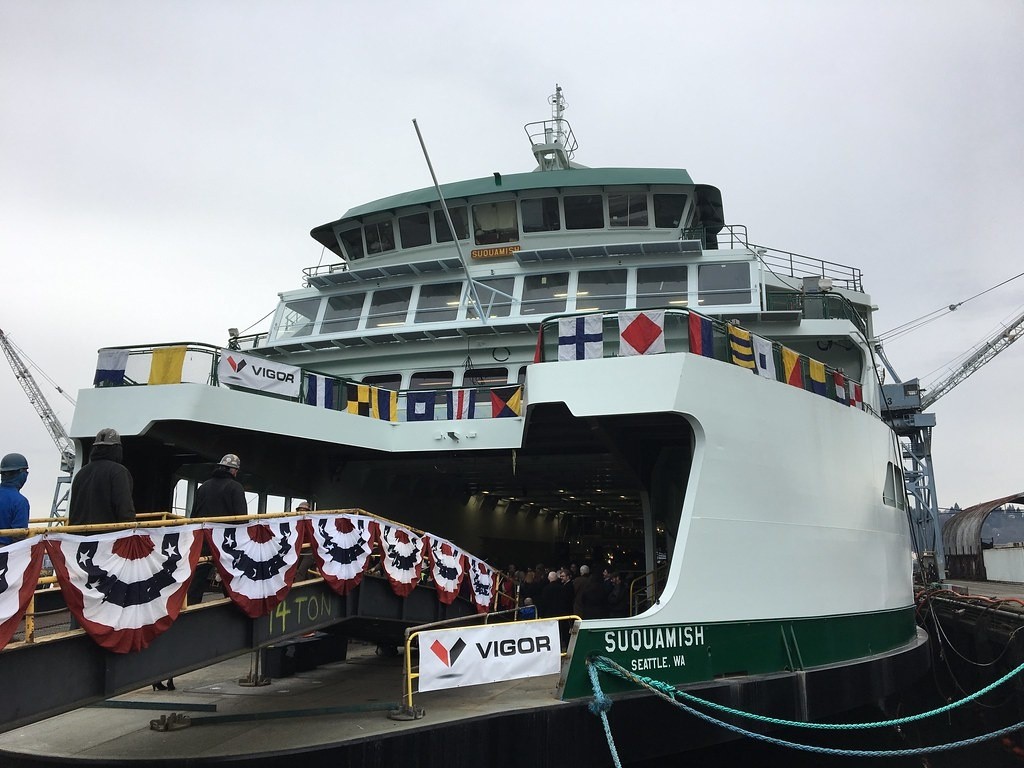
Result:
[218,454,241,470]
[296,503,311,511]
[0,453,30,473]
[93,428,121,446]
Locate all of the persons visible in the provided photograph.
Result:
[296,501,312,512]
[187,453,250,606]
[67,427,136,536]
[0,453,32,546]
[473,554,647,624]
[152,677,177,692]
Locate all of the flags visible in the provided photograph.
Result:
[306,371,523,426]
[147,346,188,384]
[418,617,563,695]
[219,348,302,398]
[93,348,129,388]
[532,307,865,412]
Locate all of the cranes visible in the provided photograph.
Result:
[873,307,1024,587]
